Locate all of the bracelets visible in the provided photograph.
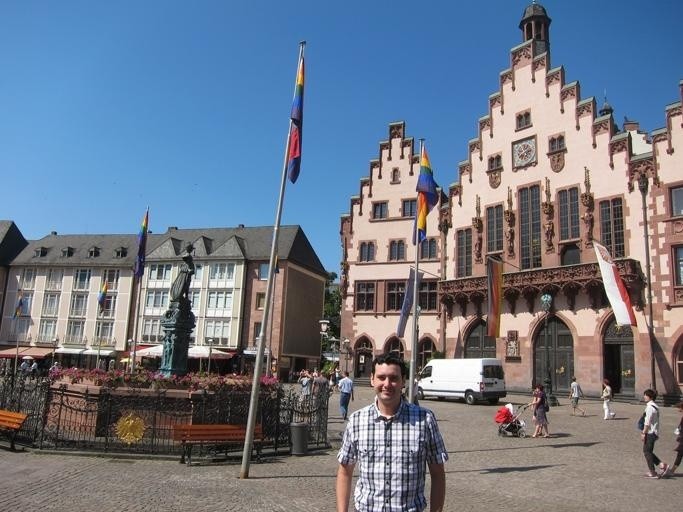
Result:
[641,431,645,434]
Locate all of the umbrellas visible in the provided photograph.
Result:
[122,345,151,356]
[187,346,232,372]
[129,343,164,359]
[21,355,34,362]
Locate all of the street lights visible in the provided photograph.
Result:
[128,339,133,371]
[51,339,57,366]
[344,338,350,372]
[541,294,558,406]
[208,339,214,373]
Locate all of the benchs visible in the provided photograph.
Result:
[0,409,29,452]
[172,422,263,467]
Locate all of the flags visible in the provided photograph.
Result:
[285,44,305,187]
[95,279,108,315]
[411,140,440,245]
[9,291,23,322]
[131,207,149,284]
[395,267,424,337]
[485,257,503,338]
[590,238,639,328]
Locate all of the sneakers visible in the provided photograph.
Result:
[643,462,670,479]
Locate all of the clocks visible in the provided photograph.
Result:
[512,134,537,172]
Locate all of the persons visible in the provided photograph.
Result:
[287,362,354,421]
[599,378,616,420]
[95,356,161,375]
[334,351,449,512]
[527,384,549,438]
[531,386,544,436]
[0,358,78,385]
[665,400,682,476]
[568,376,587,416]
[413,371,420,405]
[640,388,669,478]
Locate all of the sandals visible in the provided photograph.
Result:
[530,432,550,438]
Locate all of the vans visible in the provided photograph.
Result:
[416,358,505,405]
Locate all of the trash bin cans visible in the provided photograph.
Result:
[288,422,308,456]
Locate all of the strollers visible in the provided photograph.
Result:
[498,403,533,437]
